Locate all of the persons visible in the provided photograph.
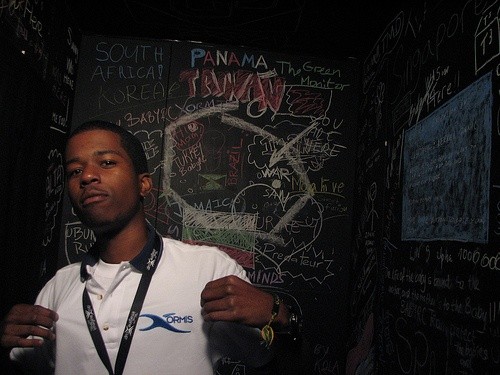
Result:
[0,120,304,375]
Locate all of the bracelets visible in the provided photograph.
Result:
[261,295,304,350]
